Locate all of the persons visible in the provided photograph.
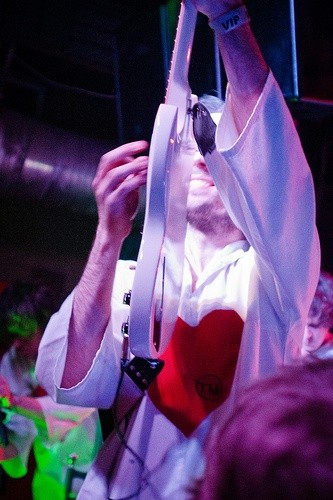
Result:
[0,0,333,500]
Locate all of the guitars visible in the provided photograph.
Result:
[121,1,201,387]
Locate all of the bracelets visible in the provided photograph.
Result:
[208,5,251,34]
